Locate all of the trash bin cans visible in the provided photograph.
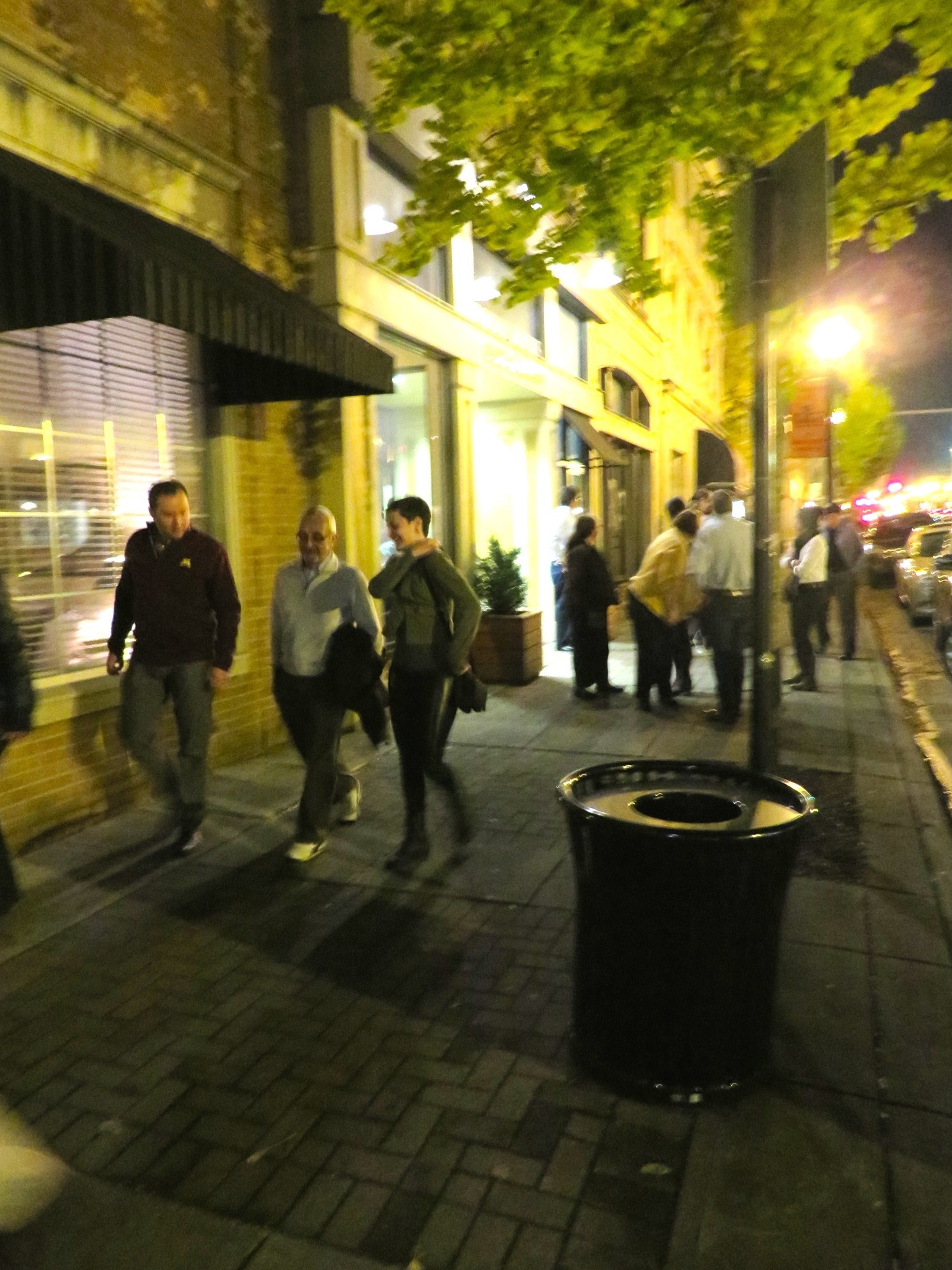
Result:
[550,759,821,1106]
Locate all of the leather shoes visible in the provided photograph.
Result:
[173,825,203,856]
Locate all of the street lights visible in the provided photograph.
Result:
[805,309,859,504]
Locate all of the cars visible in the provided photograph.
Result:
[860,511,952,678]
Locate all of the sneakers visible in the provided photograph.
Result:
[339,777,362,821]
[286,838,328,861]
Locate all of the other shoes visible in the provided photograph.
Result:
[385,836,431,876]
[453,821,476,863]
[566,622,861,729]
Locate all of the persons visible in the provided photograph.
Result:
[107,475,241,859]
[3,564,46,915]
[365,493,489,859]
[556,475,861,730]
[264,501,387,865]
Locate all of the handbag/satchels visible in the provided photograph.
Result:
[422,554,488,714]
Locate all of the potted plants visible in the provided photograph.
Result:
[469,534,543,685]
